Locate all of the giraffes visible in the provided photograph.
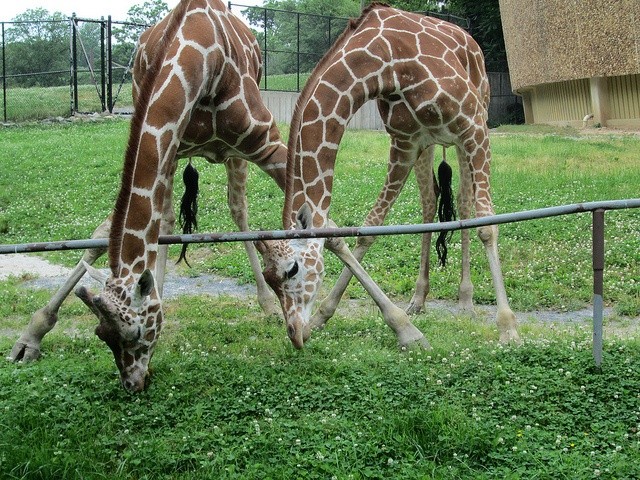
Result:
[7,0,435,397]
[253,0,523,351]
